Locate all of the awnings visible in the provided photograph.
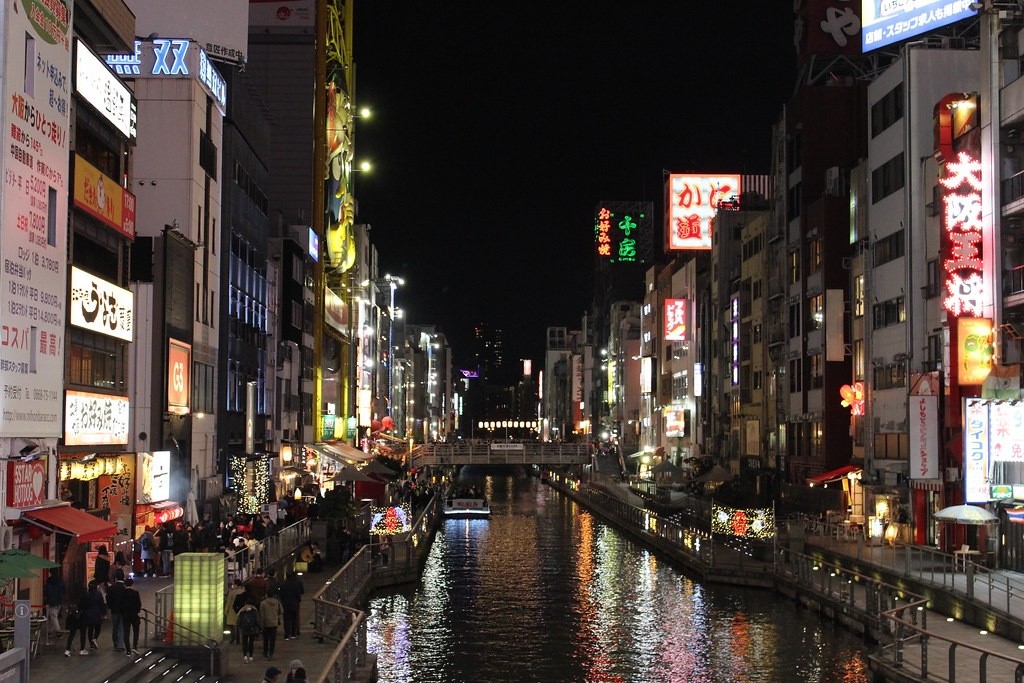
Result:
[379,433,406,442]
[368,439,392,451]
[806,466,860,485]
[22,505,117,543]
[305,445,375,465]
[0,548,61,580]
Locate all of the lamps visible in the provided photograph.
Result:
[0,452,33,465]
[327,125,349,131]
[194,240,207,249]
[839,383,864,415]
[171,219,181,232]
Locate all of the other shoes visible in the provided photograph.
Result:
[160,575,170,578]
[243,656,254,663]
[125,651,131,656]
[153,574,157,577]
[90,639,98,649]
[284,639,289,640]
[80,649,88,655]
[291,637,296,639]
[64,650,70,657]
[132,648,140,655]
[144,574,148,577]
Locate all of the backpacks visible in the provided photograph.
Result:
[142,538,152,551]
[67,598,82,621]
[114,563,124,577]
[243,614,256,633]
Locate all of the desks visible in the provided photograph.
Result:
[955,551,980,573]
[836,523,867,541]
[0,616,49,661]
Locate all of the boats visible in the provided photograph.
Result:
[442,489,490,519]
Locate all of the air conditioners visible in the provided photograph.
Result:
[281,428,292,441]
[265,429,275,441]
[198,477,212,499]
[209,474,222,497]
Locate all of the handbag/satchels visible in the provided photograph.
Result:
[169,552,174,561]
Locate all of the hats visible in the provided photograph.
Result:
[289,659,303,669]
[239,539,244,542]
[145,525,150,531]
[187,527,192,530]
[266,667,281,677]
[220,546,226,550]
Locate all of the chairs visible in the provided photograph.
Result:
[981,551,996,574]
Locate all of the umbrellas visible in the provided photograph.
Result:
[696,464,734,489]
[932,503,1000,549]
[647,459,677,480]
[326,458,397,492]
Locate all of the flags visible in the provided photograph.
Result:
[1004,507,1024,524]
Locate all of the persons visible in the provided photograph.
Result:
[225,568,301,664]
[379,535,392,569]
[339,529,352,560]
[278,486,349,531]
[298,540,324,574]
[414,432,615,457]
[447,486,478,507]
[260,659,306,683]
[65,545,141,658]
[139,512,277,579]
[395,464,459,516]
